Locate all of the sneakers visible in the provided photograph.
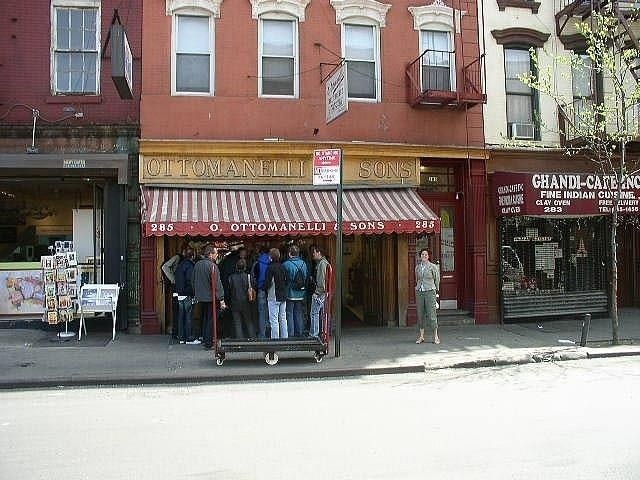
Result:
[179,337,185,344]
[186,337,202,345]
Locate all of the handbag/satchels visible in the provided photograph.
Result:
[247,273,257,304]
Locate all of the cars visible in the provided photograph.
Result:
[501,243,524,293]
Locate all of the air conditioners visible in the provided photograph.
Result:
[507,123,536,140]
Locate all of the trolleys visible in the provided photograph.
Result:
[211,265,328,367]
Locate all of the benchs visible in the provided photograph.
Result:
[498,289,611,327]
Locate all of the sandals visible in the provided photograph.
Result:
[432,335,441,345]
[415,336,424,344]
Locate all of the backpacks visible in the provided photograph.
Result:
[288,259,307,292]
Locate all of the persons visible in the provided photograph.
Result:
[415,249,441,345]
[161,241,331,349]
